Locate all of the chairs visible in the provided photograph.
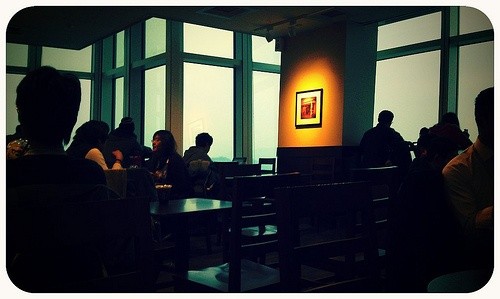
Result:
[75,158,400,293]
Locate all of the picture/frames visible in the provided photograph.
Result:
[295,88,323,127]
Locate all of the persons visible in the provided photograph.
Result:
[442,88,494,270]
[6,67,116,283]
[141,130,187,201]
[359,110,473,228]
[185,133,214,170]
[6,116,153,173]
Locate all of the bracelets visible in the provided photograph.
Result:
[113,160,122,163]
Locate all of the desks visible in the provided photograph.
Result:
[148,196,235,274]
